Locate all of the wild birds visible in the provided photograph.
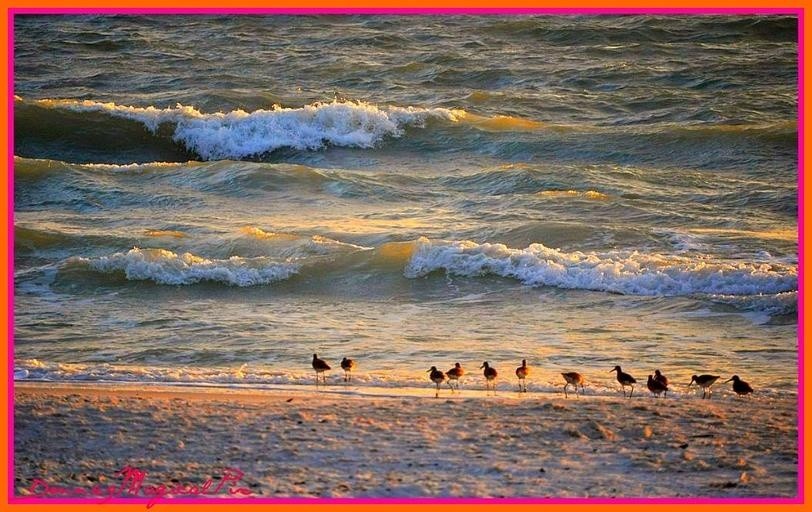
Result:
[445,363,465,390]
[480,361,500,396]
[514,357,529,393]
[647,374,668,398]
[426,365,445,398]
[560,371,587,399]
[608,365,637,400]
[688,374,721,400]
[724,375,754,400]
[653,370,667,397]
[311,354,331,387]
[340,356,355,383]
